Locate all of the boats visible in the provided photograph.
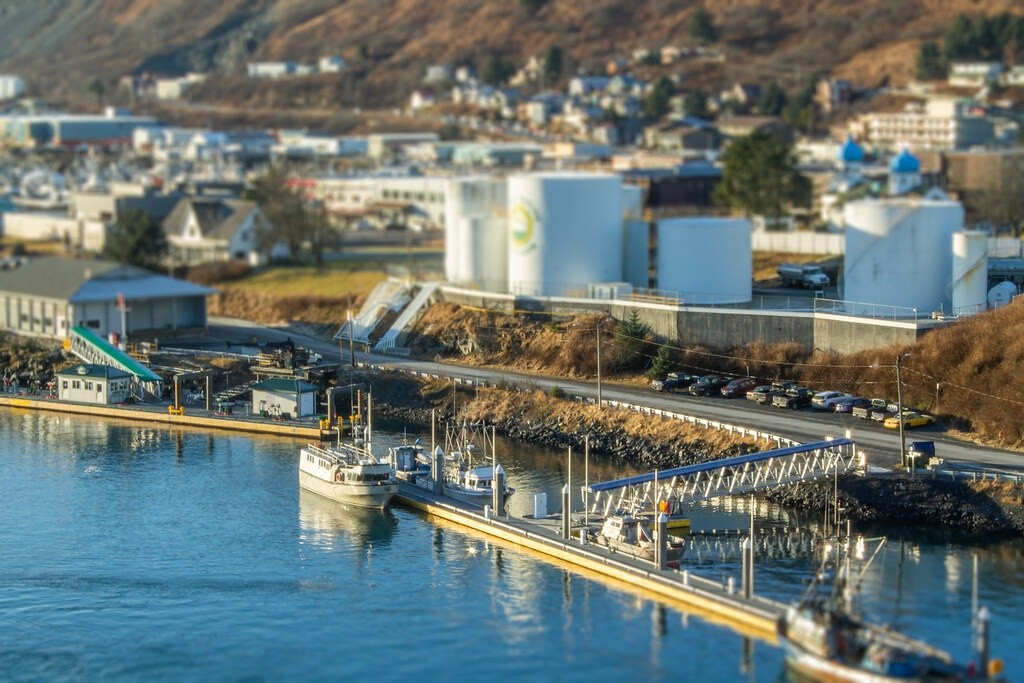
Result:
[416,442,515,515]
[775,515,1014,683]
[581,514,687,570]
[298,387,400,510]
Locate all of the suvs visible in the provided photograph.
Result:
[811,391,853,411]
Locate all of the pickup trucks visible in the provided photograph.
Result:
[871,402,909,422]
[650,369,701,392]
[853,397,893,419]
[688,374,734,397]
[754,381,799,405]
[772,387,821,410]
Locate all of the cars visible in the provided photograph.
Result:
[297,347,323,366]
[720,379,759,397]
[836,396,873,413]
[883,411,937,429]
[746,385,772,400]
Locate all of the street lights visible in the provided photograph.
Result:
[223,371,234,414]
[597,315,614,409]
[895,351,913,466]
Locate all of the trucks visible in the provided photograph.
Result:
[776,264,830,291]
